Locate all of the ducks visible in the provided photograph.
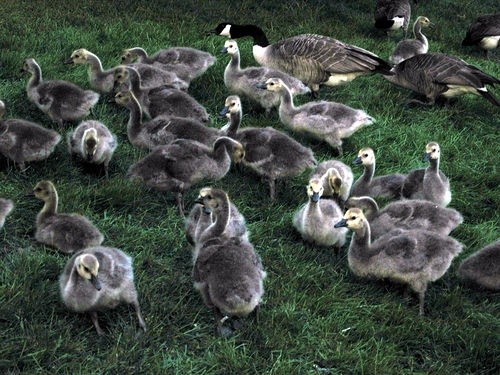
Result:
[0,0,500,337]
[334,207,467,322]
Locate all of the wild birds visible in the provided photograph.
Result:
[381,51,500,107]
[210,22,397,92]
